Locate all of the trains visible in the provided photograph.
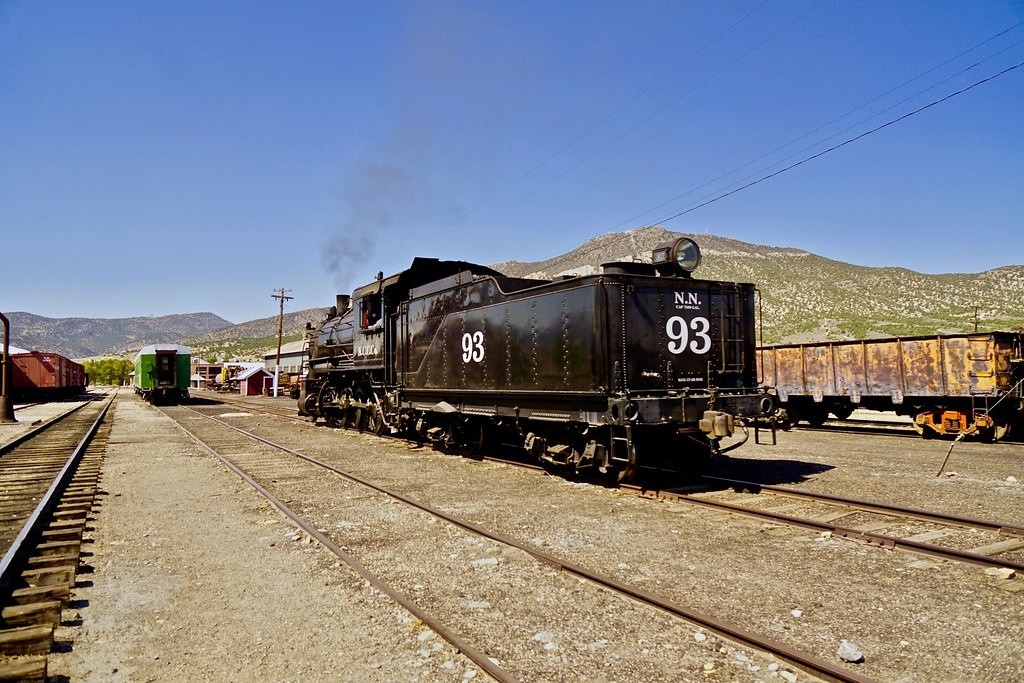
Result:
[289,237,781,483]
[133,344,190,403]
